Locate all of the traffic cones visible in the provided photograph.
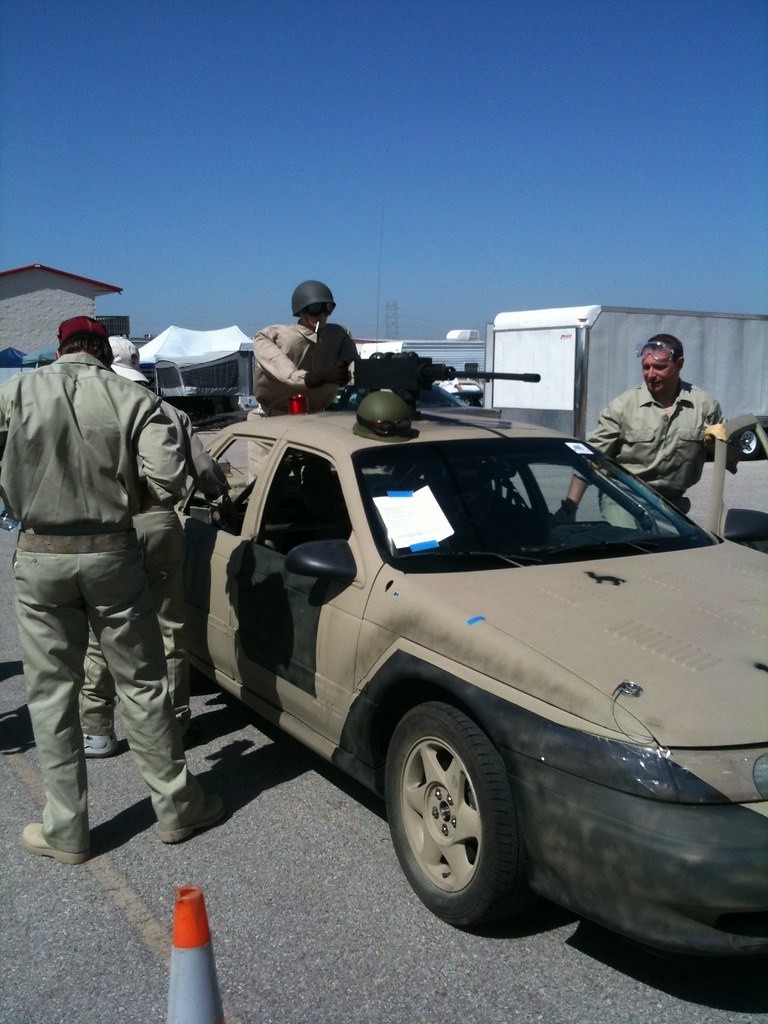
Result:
[168,888,225,1024]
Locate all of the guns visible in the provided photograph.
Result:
[339,352,541,400]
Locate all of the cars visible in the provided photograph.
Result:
[184,408,768,957]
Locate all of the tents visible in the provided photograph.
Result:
[138,325,253,366]
[0,343,58,372]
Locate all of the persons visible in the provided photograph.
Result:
[555,333,727,530]
[0,316,188,863]
[248,280,353,485]
[77,336,232,758]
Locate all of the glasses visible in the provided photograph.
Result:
[375,418,412,436]
[634,342,674,361]
[306,302,334,317]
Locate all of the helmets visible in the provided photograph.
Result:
[291,281,336,317]
[353,392,415,443]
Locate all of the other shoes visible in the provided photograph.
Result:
[80,732,118,758]
[158,793,226,844]
[24,823,91,863]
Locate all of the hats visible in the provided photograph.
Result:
[106,336,149,383]
[55,316,110,348]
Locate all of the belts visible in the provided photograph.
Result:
[16,527,137,554]
[139,496,175,513]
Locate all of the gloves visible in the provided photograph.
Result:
[543,499,578,535]
[304,361,350,390]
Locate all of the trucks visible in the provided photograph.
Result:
[360,303,768,543]
